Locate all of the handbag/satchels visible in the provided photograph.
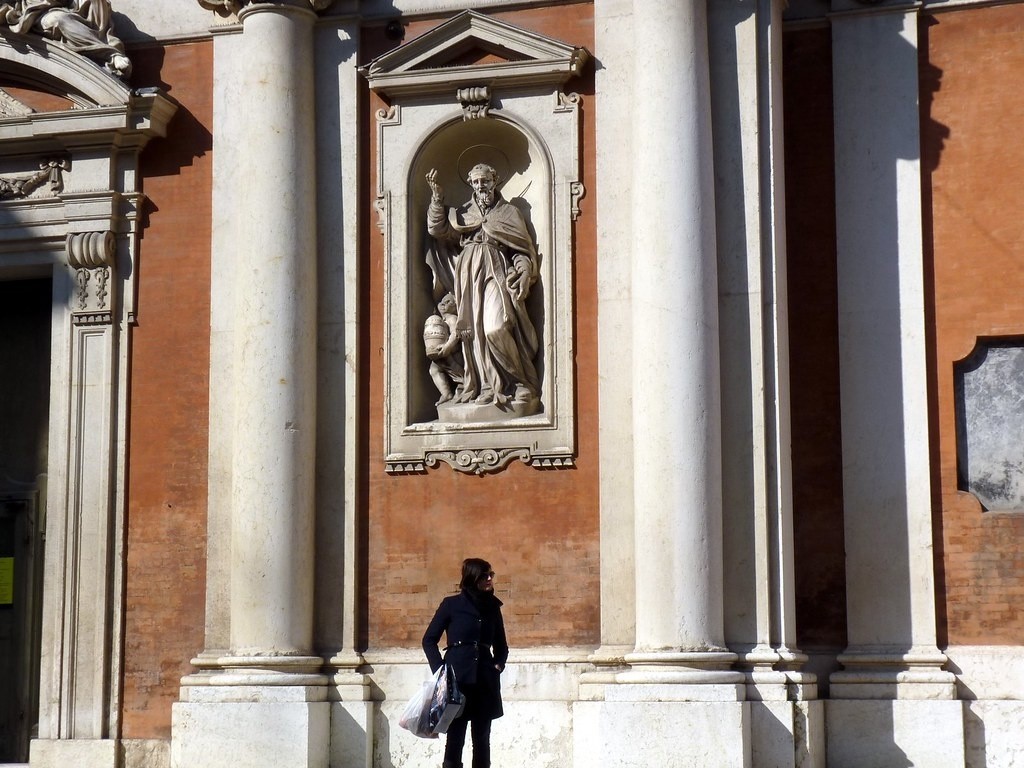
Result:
[399,664,467,738]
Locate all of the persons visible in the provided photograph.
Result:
[422,558,509,768]
[424,163,538,404]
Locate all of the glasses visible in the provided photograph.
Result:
[482,571,495,581]
